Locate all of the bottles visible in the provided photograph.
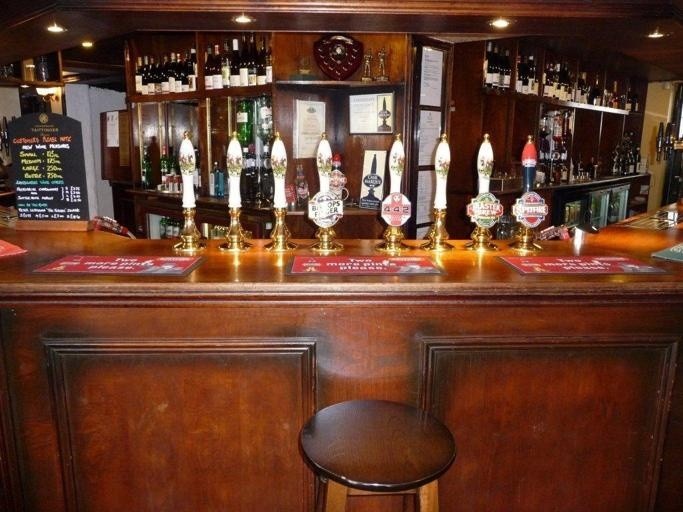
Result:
[266,43,273,83]
[553,64,560,97]
[142,56,148,95]
[571,150,599,184]
[209,161,218,197]
[500,47,505,90]
[610,132,641,176]
[188,49,197,91]
[194,147,199,191]
[260,146,272,201]
[198,165,201,195]
[235,97,252,148]
[543,63,549,98]
[161,145,170,185]
[492,46,500,89]
[293,164,311,208]
[168,53,176,92]
[567,84,572,101]
[486,42,494,88]
[257,47,266,85]
[576,71,602,105]
[204,48,212,90]
[504,49,512,92]
[248,33,257,86]
[571,80,575,101]
[564,84,568,101]
[215,169,224,198]
[558,61,570,87]
[25,65,35,81]
[37,55,49,82]
[528,55,534,93]
[555,84,561,100]
[142,144,153,189]
[221,40,231,89]
[240,147,249,201]
[559,83,564,100]
[230,39,240,88]
[540,109,571,185]
[239,34,249,87]
[160,216,166,239]
[185,53,190,63]
[256,94,273,145]
[603,80,626,109]
[135,57,142,95]
[175,54,182,92]
[166,217,172,238]
[170,162,177,176]
[522,55,528,94]
[543,72,546,95]
[516,55,522,93]
[181,59,189,92]
[533,79,539,96]
[548,63,554,98]
[247,144,258,201]
[155,55,165,94]
[211,45,223,89]
[331,151,346,199]
[626,87,639,113]
[148,57,155,95]
[173,218,180,237]
[161,61,169,94]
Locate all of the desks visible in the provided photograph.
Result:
[1,198,683,512]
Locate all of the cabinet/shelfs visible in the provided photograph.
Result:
[274,31,412,238]
[124,31,273,238]
[442,30,650,239]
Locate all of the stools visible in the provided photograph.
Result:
[299,398,455,512]
